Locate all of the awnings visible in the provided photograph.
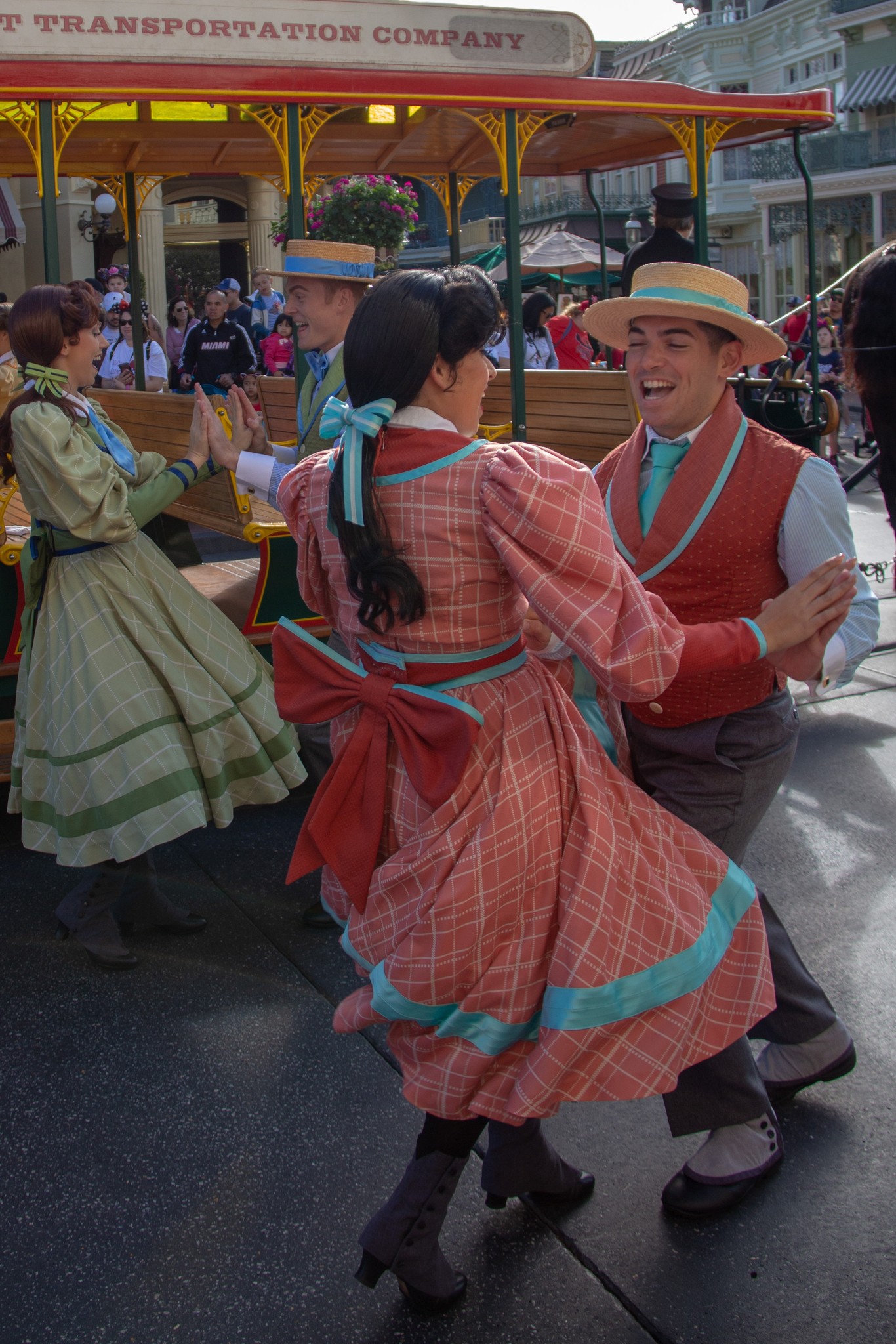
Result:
[0,176,26,251]
[836,64,896,113]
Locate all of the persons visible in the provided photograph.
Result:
[622,182,711,297]
[273,261,857,1300]
[250,265,286,376]
[0,302,25,419]
[524,265,881,1215]
[0,284,307,962]
[738,288,847,466]
[243,290,260,304]
[260,313,293,377]
[213,278,255,340]
[66,264,167,392]
[178,290,257,392]
[236,368,269,440]
[196,240,388,495]
[166,297,201,366]
[479,290,627,370]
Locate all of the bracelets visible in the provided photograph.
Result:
[126,384,129,390]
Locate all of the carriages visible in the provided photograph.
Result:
[0,0,896,652]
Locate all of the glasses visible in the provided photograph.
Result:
[789,305,798,308]
[222,290,235,296]
[119,318,132,326]
[173,306,189,312]
[542,309,553,318]
[830,296,844,303]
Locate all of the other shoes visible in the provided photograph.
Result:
[836,444,847,455]
[839,423,856,439]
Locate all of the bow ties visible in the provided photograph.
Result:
[304,351,329,382]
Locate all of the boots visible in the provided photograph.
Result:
[354,1131,467,1298]
[481,1117,594,1209]
[55,865,138,969]
[111,848,207,937]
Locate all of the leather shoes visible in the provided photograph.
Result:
[660,1158,783,1214]
[759,1041,858,1107]
[302,901,338,930]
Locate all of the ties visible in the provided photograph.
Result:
[88,407,135,476]
[638,440,693,542]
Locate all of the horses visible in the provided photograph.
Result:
[825,239,895,537]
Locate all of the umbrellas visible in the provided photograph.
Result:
[463,223,625,293]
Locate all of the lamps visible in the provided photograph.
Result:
[78,194,116,241]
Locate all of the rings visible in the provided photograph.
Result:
[111,385,112,387]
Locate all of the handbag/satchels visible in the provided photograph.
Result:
[168,365,181,389]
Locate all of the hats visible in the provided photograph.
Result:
[582,261,788,365]
[213,278,241,292]
[103,292,124,311]
[256,239,380,283]
[651,182,695,219]
[244,290,260,303]
[830,288,846,297]
[816,295,825,302]
[786,296,804,305]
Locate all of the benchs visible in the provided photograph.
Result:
[0,366,639,564]
